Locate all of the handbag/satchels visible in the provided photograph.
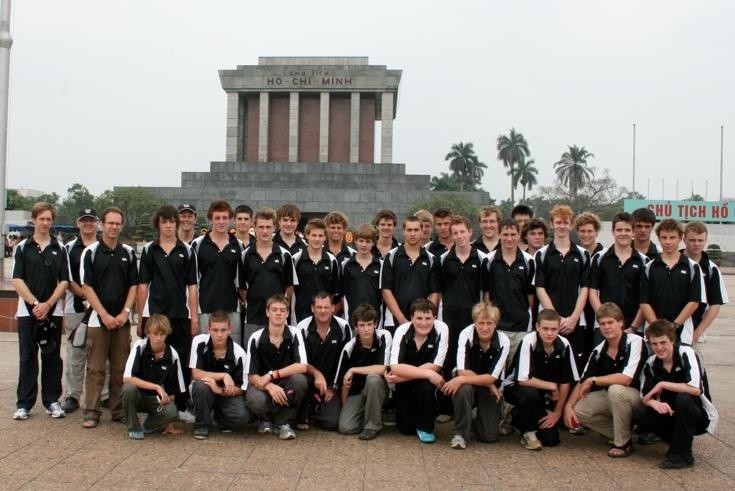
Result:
[67,306,94,348]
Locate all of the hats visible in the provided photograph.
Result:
[177,203,198,216]
[77,207,97,220]
[34,315,57,355]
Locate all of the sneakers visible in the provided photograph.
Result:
[216,422,233,433]
[193,427,209,441]
[116,416,128,424]
[129,431,145,440]
[62,398,80,412]
[498,401,514,437]
[296,422,310,430]
[177,411,196,425]
[141,426,158,434]
[81,416,98,427]
[437,414,451,424]
[451,434,466,449]
[520,431,543,452]
[416,430,436,444]
[607,439,633,458]
[471,407,477,417]
[661,451,694,470]
[359,427,377,440]
[568,425,587,436]
[274,425,296,440]
[102,400,110,406]
[13,408,29,420]
[638,433,662,445]
[45,403,66,418]
[257,419,272,434]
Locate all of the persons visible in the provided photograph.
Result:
[246,295,310,440]
[386,298,449,444]
[290,219,347,328]
[273,205,306,257]
[323,211,356,272]
[230,204,254,249]
[4,232,25,258]
[425,209,454,256]
[472,205,503,262]
[522,219,549,258]
[80,207,138,428]
[370,210,403,262]
[574,212,604,257]
[677,222,729,374]
[564,302,648,460]
[380,216,440,328]
[637,320,719,472]
[237,208,298,350]
[134,205,200,429]
[50,228,77,246]
[10,202,66,417]
[511,204,533,250]
[189,311,248,442]
[118,313,186,442]
[295,292,353,436]
[631,208,661,262]
[447,303,511,449]
[531,204,590,437]
[480,218,536,436]
[59,207,114,413]
[340,224,387,334]
[639,218,705,451]
[176,203,198,248]
[437,217,486,424]
[190,200,244,357]
[500,309,579,458]
[334,304,393,440]
[590,212,650,343]
[414,209,435,249]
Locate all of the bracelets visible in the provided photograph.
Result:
[47,302,52,310]
[276,370,281,380]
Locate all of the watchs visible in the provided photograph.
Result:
[123,308,132,314]
[674,322,681,329]
[631,326,639,332]
[31,301,39,308]
[592,376,597,385]
[269,370,274,380]
